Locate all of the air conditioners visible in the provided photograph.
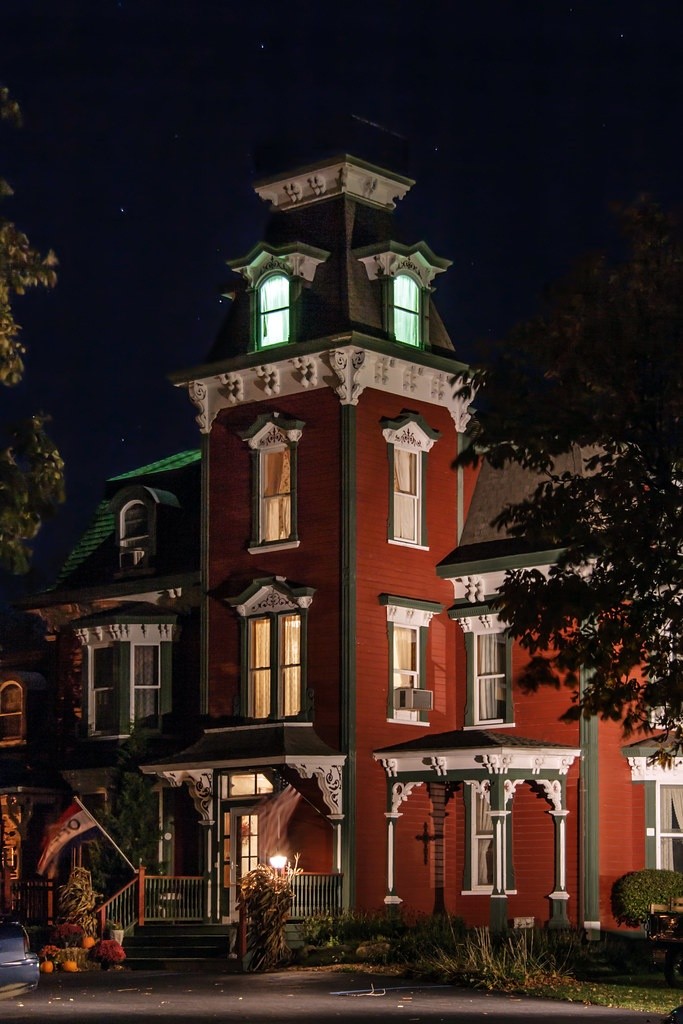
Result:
[119,551,144,567]
[393,687,434,711]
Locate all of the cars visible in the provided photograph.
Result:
[0,920,40,1002]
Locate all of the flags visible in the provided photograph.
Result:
[252,778,302,849]
[36,799,97,875]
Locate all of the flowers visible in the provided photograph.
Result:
[33,921,131,976]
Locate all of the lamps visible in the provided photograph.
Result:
[266,846,292,874]
